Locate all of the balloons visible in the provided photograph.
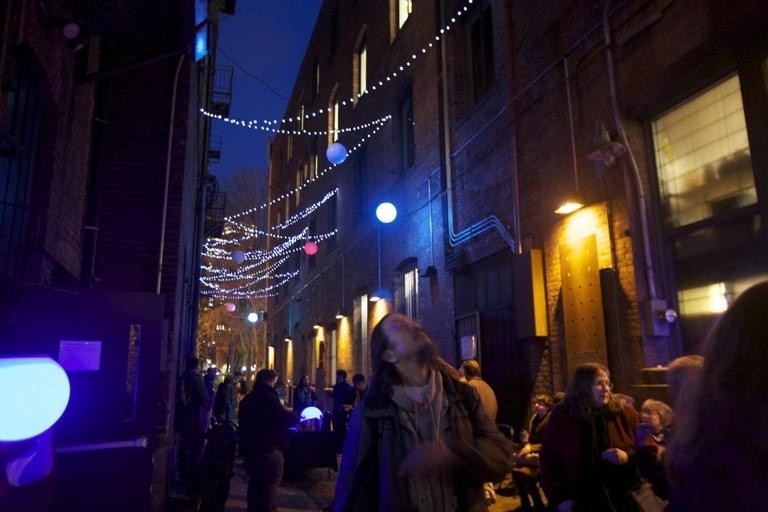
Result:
[231,250,245,265]
[305,241,319,255]
[326,143,348,166]
[225,303,236,313]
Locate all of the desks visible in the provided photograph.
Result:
[286,429,334,475]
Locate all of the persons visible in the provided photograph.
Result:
[666,280,768,512]
[177,358,498,510]
[334,313,513,512]
[514,354,704,511]
